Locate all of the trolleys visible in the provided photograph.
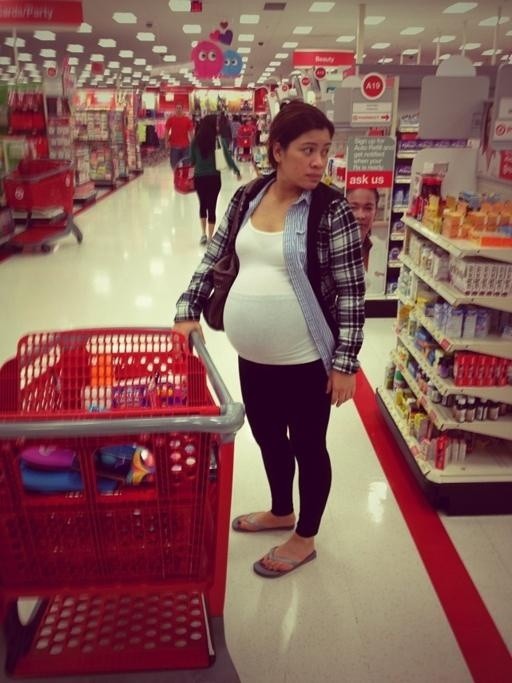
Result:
[235,128,254,162]
[0,326,243,682]
[2,156,82,255]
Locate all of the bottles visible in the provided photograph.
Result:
[417,357,509,423]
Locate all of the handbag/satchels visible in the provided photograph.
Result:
[203,249,240,331]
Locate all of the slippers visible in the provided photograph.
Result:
[252,543,316,579]
[232,511,295,533]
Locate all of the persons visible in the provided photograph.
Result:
[164,102,256,245]
[173,100,366,578]
[347,187,389,296]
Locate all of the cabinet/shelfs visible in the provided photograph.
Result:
[376,124,509,515]
[50,103,127,198]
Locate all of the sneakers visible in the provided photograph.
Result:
[199,235,207,244]
